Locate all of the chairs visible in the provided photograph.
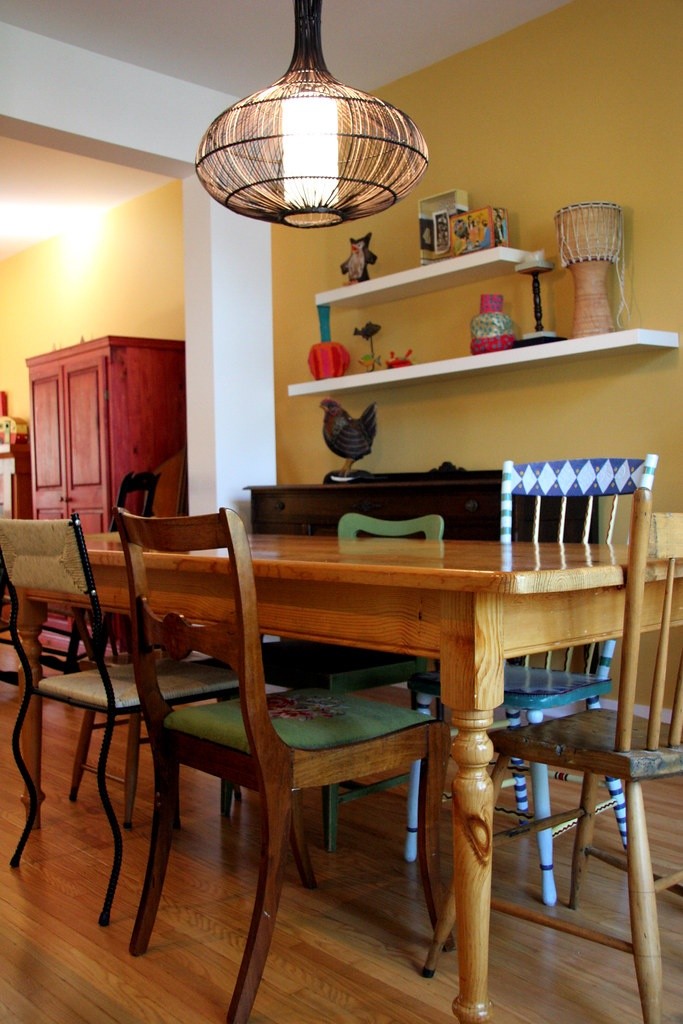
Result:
[0,456,683,1024]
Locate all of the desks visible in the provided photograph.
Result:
[15,532,683,1024]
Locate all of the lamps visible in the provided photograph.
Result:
[194,0,430,229]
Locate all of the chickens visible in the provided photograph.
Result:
[318,398,377,479]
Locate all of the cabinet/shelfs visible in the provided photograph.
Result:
[243,479,599,676]
[25,334,188,660]
[285,245,680,398]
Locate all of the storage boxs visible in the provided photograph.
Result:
[448,205,509,259]
[418,187,468,267]
